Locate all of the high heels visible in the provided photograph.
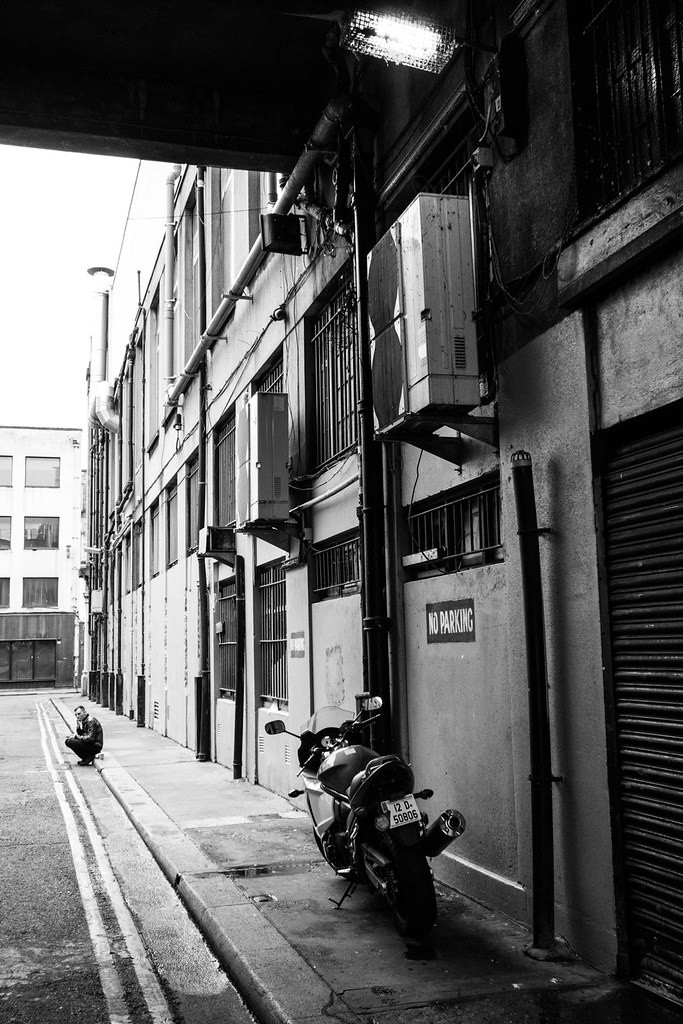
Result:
[79,754,95,766]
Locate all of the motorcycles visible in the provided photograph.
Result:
[264,693,467,944]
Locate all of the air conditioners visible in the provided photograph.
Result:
[196,526,236,556]
[229,391,289,531]
[91,589,103,613]
[364,193,482,441]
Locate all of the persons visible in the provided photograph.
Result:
[64,706,103,765]
